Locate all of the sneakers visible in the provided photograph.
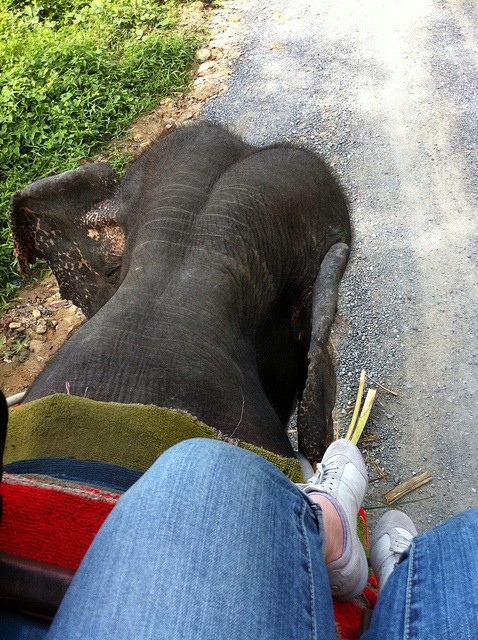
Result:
[295,438,368,602]
[370,509,418,594]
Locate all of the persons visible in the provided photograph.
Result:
[47,439,477,635]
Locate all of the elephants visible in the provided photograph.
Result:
[9,120,353,461]
